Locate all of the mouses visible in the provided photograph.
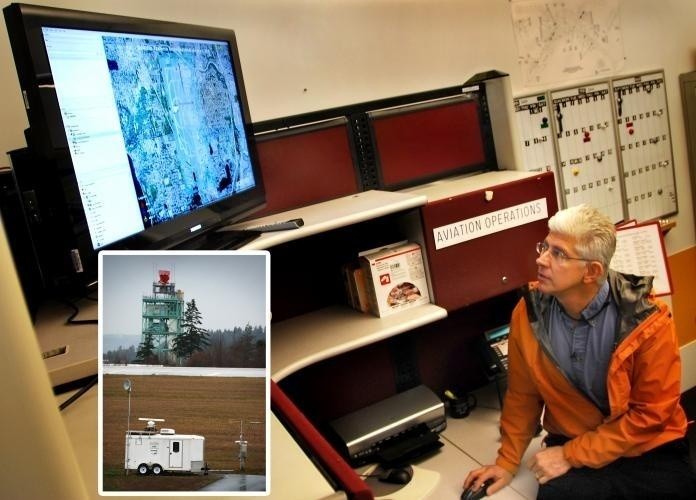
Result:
[375,460,416,485]
[460,478,491,500]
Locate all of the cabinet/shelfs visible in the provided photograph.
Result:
[33,184,448,392]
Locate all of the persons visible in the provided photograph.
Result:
[462,204,696,500]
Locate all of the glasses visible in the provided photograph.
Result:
[536,242,592,262]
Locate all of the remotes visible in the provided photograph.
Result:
[244,216,305,232]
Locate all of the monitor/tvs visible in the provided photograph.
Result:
[1,0,262,294]
